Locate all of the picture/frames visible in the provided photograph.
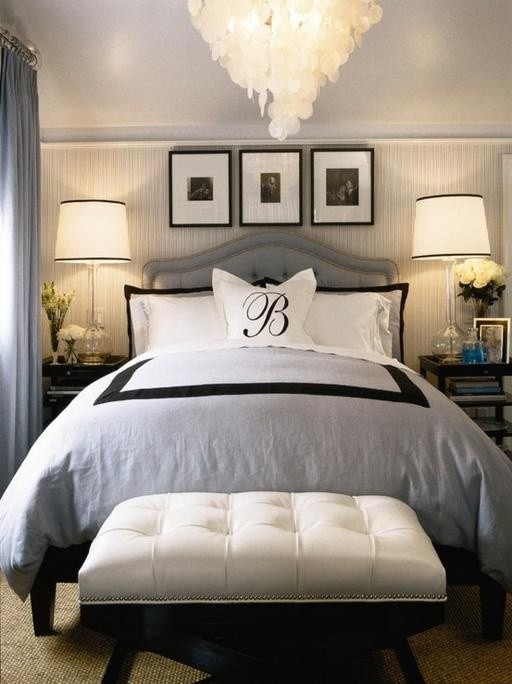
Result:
[311,148,374,225]
[169,150,232,227]
[473,317,510,364]
[239,149,303,226]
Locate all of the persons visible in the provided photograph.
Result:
[347,179,357,204]
[263,174,277,202]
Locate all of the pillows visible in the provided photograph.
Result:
[121,265,410,366]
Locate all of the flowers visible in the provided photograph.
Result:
[453,257,510,319]
[39,282,76,351]
[56,324,86,362]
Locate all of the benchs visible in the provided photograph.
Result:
[77,491,448,683]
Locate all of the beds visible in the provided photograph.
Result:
[29,231,507,639]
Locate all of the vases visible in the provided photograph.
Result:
[48,320,61,365]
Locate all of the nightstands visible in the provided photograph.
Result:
[418,355,512,449]
[41,353,131,431]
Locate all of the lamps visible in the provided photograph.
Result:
[52,199,132,367]
[187,0,383,141]
[411,193,491,361]
